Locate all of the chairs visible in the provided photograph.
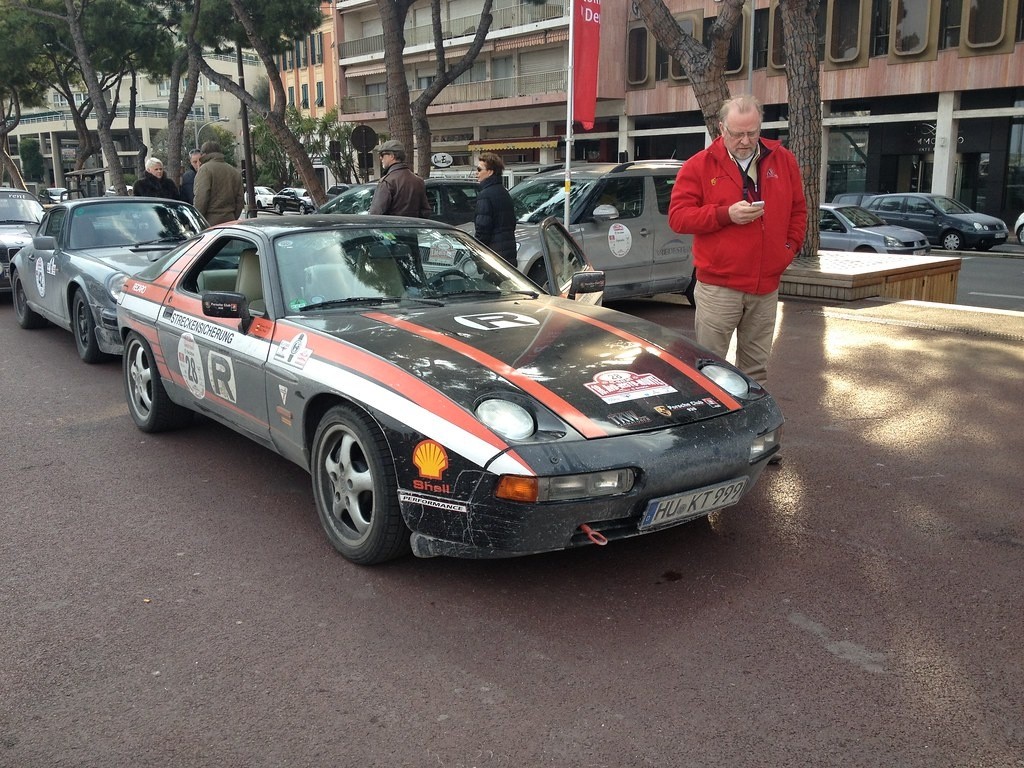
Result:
[236,248,308,312]
[339,243,408,299]
[442,30,452,39]
[457,26,476,36]
[66,217,100,250]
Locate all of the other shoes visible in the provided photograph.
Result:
[767,454,782,466]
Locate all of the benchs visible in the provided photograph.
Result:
[197,269,242,296]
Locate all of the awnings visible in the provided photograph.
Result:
[467,137,561,151]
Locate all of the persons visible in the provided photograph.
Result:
[192,140,244,227]
[669,94,806,387]
[134,157,179,199]
[474,152,518,269]
[183,148,203,205]
[371,140,432,219]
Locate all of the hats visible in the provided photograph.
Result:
[378,140,405,152]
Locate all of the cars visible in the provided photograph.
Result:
[0,184,53,300]
[271,187,318,215]
[862,190,1006,248]
[8,196,216,363]
[1015,210,1024,244]
[325,183,361,204]
[815,202,930,255]
[832,192,879,206]
[452,159,699,309]
[244,186,277,208]
[312,166,538,228]
[114,213,793,566]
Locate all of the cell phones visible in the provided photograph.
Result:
[752,201,765,208]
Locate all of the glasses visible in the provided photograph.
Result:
[189,149,200,157]
[476,167,487,172]
[380,152,390,158]
[726,127,759,140]
